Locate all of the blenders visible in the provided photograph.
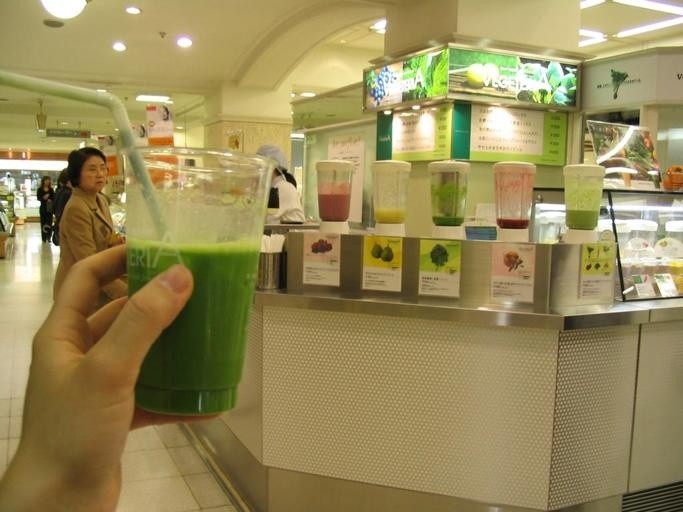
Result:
[315,158,607,247]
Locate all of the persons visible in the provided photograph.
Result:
[37,176,55,242]
[53,167,73,246]
[1,241,194,512]
[54,178,63,215]
[54,145,125,306]
[256,145,304,223]
[1,172,16,195]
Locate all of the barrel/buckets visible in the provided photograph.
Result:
[257,250,288,290]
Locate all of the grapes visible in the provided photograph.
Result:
[369,66,396,105]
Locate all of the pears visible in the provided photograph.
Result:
[370,241,382,258]
[380,243,393,262]
[467,73,485,89]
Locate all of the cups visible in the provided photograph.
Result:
[122,148,278,419]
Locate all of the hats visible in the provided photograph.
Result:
[256,144,288,171]
